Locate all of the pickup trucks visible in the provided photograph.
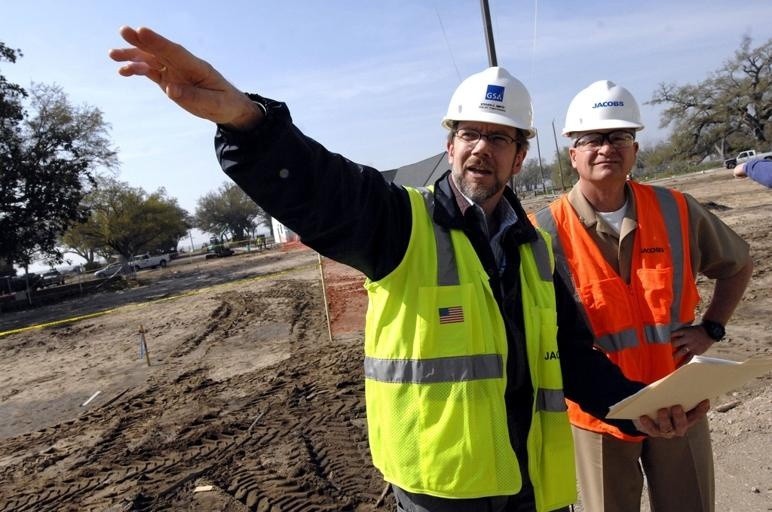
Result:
[724,149,772,169]
[127,253,171,272]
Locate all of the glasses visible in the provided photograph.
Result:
[573,130,640,152]
[451,126,522,148]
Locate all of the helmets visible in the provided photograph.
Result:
[564,77,644,137]
[440,63,538,141]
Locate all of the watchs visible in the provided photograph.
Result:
[698,318,726,342]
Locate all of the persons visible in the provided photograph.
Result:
[107,24,710,512]
[732,156,772,190]
[524,77,752,511]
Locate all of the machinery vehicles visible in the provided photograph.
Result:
[206,231,232,260]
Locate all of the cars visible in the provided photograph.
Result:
[0,269,66,295]
[93,261,125,280]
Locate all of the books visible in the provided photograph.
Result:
[605,351,772,425]
